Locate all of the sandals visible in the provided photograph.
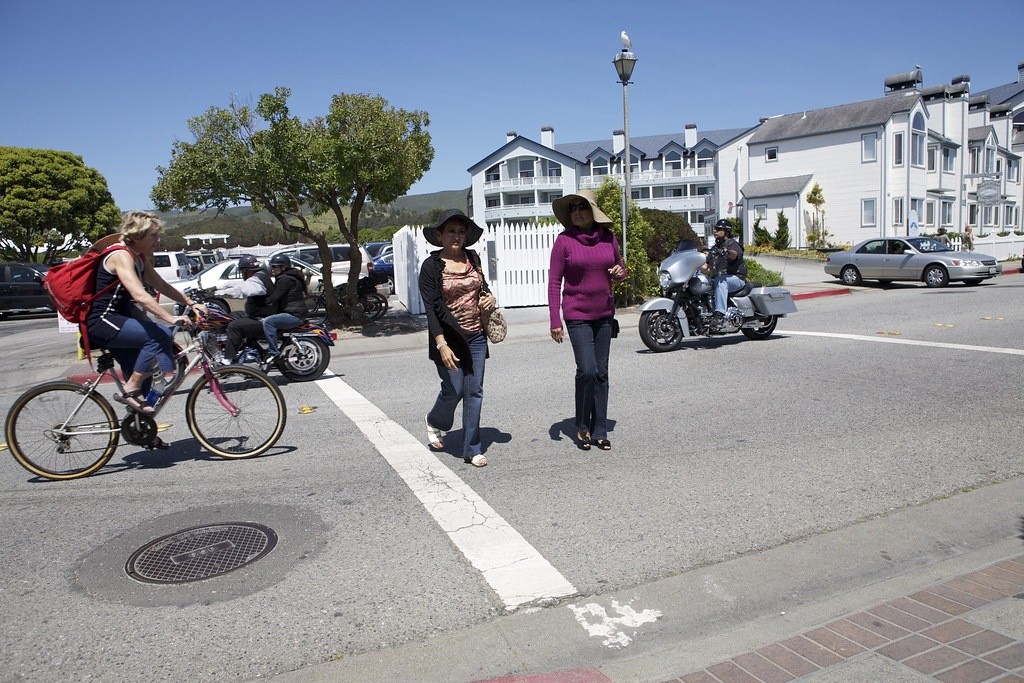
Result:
[114,386,156,416]
[592,437,612,451]
[575,431,593,450]
[144,434,171,450]
[463,452,487,467]
[425,414,444,448]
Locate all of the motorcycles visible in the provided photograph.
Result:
[638,238,797,353]
[173,287,337,382]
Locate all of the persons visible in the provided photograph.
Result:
[418,208,497,467]
[548,190,626,451]
[961,226,974,252]
[203,254,309,367]
[889,243,904,254]
[933,227,952,247]
[700,219,746,314]
[78,211,209,450]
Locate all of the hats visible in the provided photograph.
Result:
[552,189,614,230]
[86,233,123,253]
[423,209,484,247]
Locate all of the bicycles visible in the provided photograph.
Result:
[301,278,389,326]
[5,308,288,482]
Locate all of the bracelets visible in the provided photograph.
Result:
[436,341,447,350]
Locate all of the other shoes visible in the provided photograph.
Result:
[260,352,281,365]
[217,360,235,379]
[710,314,725,326]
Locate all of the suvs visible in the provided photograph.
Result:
[150,241,395,293]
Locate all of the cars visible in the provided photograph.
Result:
[823,236,1003,288]
[0,262,58,313]
[155,257,324,315]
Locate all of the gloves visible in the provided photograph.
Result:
[716,248,730,256]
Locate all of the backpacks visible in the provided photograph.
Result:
[43,245,146,323]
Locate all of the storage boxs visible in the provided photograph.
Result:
[749,287,798,316]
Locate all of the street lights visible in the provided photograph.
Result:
[612,47,639,308]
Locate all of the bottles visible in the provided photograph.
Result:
[145,379,167,408]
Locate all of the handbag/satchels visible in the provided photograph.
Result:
[611,319,620,337]
[469,249,508,343]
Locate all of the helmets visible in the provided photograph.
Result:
[269,254,289,266]
[237,254,260,269]
[714,219,732,230]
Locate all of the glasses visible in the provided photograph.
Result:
[440,228,467,236]
[567,202,590,213]
[713,227,725,232]
